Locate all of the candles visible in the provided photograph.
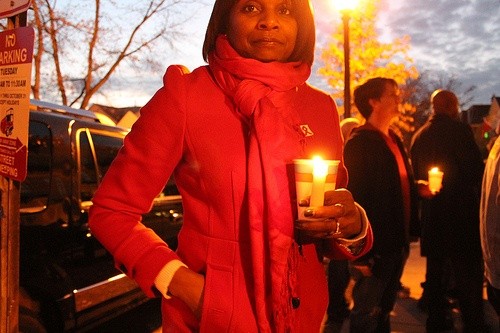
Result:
[310,155,329,208]
[429,166,444,193]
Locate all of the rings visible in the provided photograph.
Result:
[333,222,341,235]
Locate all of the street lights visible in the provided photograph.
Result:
[336,0,359,118]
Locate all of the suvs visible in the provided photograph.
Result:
[18,100,183,333]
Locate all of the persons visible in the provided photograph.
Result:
[87,0,374,333]
[325,77,500,333]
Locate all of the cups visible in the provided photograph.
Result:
[293,158,341,221]
[428,170,444,194]
